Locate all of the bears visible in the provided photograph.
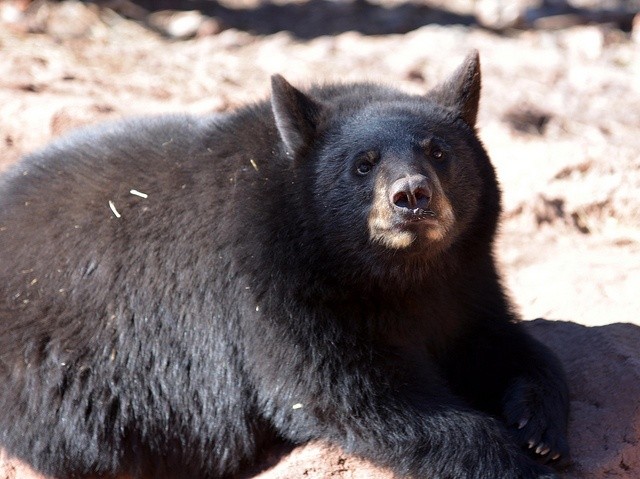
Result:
[0,47,577,479]
